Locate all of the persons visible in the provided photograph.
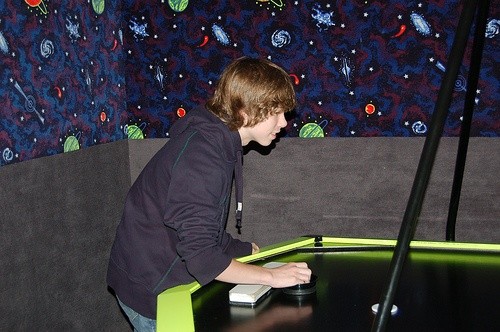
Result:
[104,56,314,332]
